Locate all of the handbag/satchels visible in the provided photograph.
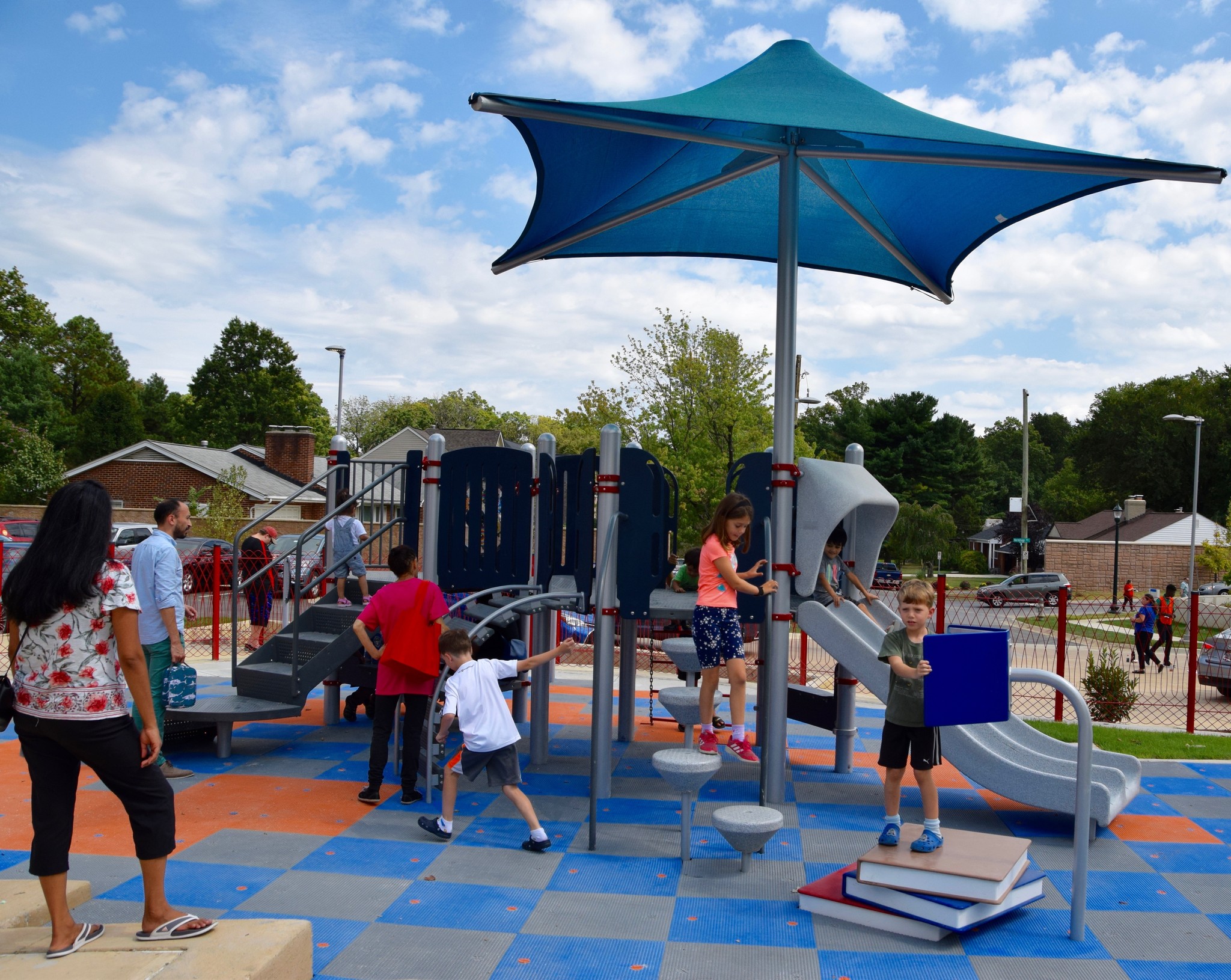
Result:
[0,675,14,732]
[159,662,197,709]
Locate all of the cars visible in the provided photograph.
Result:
[0,516,325,634]
[1198,580,1231,595]
[1197,627,1231,696]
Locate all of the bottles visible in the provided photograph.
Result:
[1131,650,1135,663]
[1126,655,1130,662]
[1169,663,1173,672]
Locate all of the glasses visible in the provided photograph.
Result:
[269,535,272,543]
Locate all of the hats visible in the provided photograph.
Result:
[262,526,278,545]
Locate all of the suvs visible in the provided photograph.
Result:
[976,572,1072,608]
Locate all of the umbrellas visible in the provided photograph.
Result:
[463,38,1227,819]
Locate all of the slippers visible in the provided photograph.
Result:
[910,829,944,853]
[417,816,452,839]
[522,834,551,851]
[712,716,726,728]
[885,619,901,635]
[46,923,105,959]
[878,823,900,845]
[678,723,685,732]
[135,913,218,941]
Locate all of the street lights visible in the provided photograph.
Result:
[1107,503,1123,614]
[325,345,346,435]
[1162,414,1204,644]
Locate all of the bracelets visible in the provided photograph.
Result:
[753,585,763,597]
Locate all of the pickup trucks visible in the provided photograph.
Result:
[870,562,903,591]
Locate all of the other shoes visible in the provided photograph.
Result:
[663,620,691,631]
[1157,664,1163,673]
[1163,661,1177,668]
[1144,654,1150,666]
[1122,610,1126,612]
[244,643,262,653]
[1132,670,1145,674]
[1131,610,1135,612]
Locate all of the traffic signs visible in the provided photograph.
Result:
[1013,538,1030,546]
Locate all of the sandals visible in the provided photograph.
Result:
[362,594,372,606]
[337,597,352,607]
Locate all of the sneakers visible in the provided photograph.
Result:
[358,788,380,802]
[725,735,760,763]
[400,786,423,804]
[159,760,195,780]
[697,729,719,755]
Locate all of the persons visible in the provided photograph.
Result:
[877,579,935,854]
[690,491,778,765]
[0,478,216,959]
[665,546,700,593]
[242,526,278,653]
[1122,579,1136,612]
[418,628,577,848]
[1180,578,1189,598]
[322,491,372,607]
[1130,593,1164,674]
[814,527,900,636]
[1144,584,1177,668]
[343,544,453,804]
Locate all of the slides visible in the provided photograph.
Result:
[790,598,1144,826]
